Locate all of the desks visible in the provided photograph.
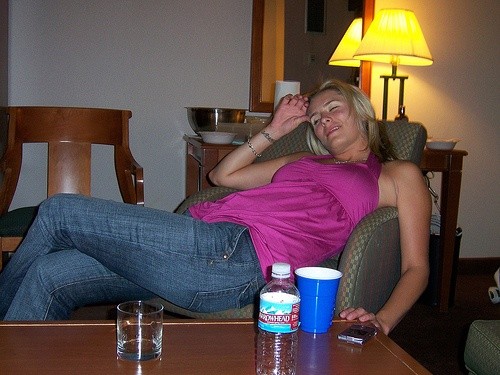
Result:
[184,135,467,315]
[0,317,433,375]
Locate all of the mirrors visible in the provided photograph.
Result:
[250,0,375,110]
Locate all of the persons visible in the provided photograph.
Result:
[0,79,432,336]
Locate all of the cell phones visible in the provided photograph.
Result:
[337,323,378,345]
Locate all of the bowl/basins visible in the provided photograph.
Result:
[197,132,238,144]
[184,106,250,137]
[427,138,459,150]
[218,123,263,142]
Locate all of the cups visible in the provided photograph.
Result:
[274,80,300,115]
[116,301,164,363]
[294,266,344,334]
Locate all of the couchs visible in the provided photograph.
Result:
[143,119,428,325]
[464,320,500,375]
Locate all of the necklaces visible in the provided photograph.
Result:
[333,156,368,165]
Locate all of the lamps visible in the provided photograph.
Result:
[328,19,362,82]
[352,9,435,119]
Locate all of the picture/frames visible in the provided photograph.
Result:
[306,0,328,34]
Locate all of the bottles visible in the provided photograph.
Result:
[256,262,301,375]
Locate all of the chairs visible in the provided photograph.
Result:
[0,107,144,274]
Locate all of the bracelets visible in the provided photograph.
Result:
[247,139,262,158]
[260,130,277,144]
